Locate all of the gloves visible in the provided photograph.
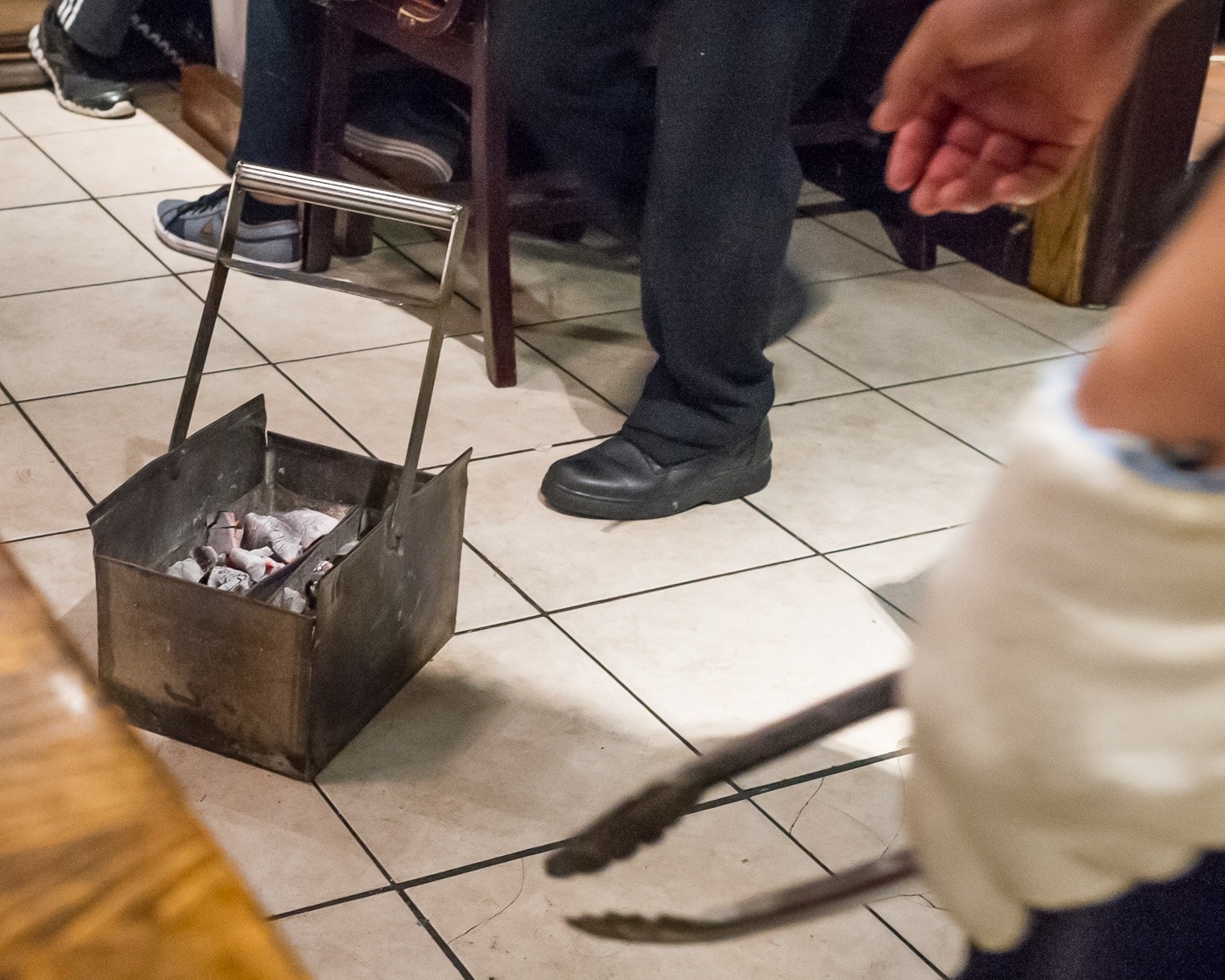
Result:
[904,335,1225,951]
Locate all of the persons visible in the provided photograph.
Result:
[28,0,209,118]
[534,0,850,520]
[864,0,1225,980]
[153,0,471,273]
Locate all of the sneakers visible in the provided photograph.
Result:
[541,418,772,520]
[765,277,808,346]
[343,111,456,182]
[27,8,134,117]
[154,185,302,277]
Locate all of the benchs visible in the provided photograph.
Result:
[298,1,946,385]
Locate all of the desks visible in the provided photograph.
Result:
[1,537,303,980]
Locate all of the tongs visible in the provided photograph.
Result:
[544,661,923,945]
